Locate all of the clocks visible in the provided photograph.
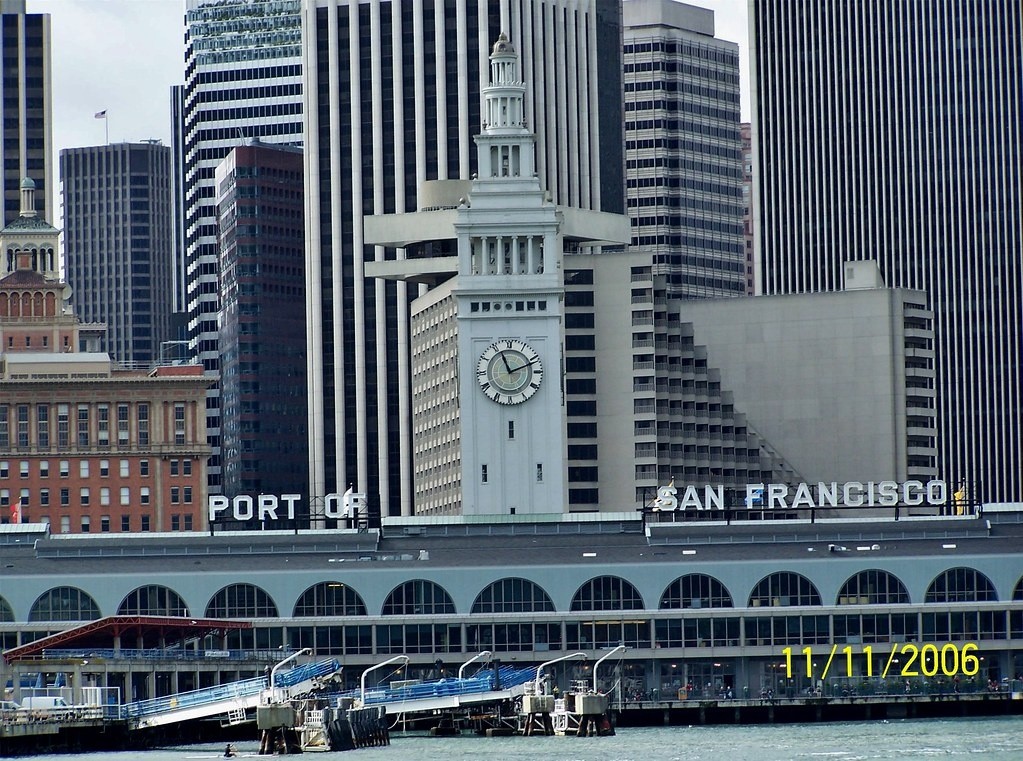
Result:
[478,339,542,406]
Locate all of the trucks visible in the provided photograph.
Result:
[23,696,68,709]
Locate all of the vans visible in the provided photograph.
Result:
[0,700,22,712]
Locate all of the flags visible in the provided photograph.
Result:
[95,111,105,118]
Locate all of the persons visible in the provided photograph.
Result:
[718,684,733,699]
[987,677,999,692]
[906,680,910,692]
[687,682,693,699]
[597,689,608,697]
[761,686,774,699]
[954,676,960,692]
[809,685,823,697]
[225,744,238,757]
